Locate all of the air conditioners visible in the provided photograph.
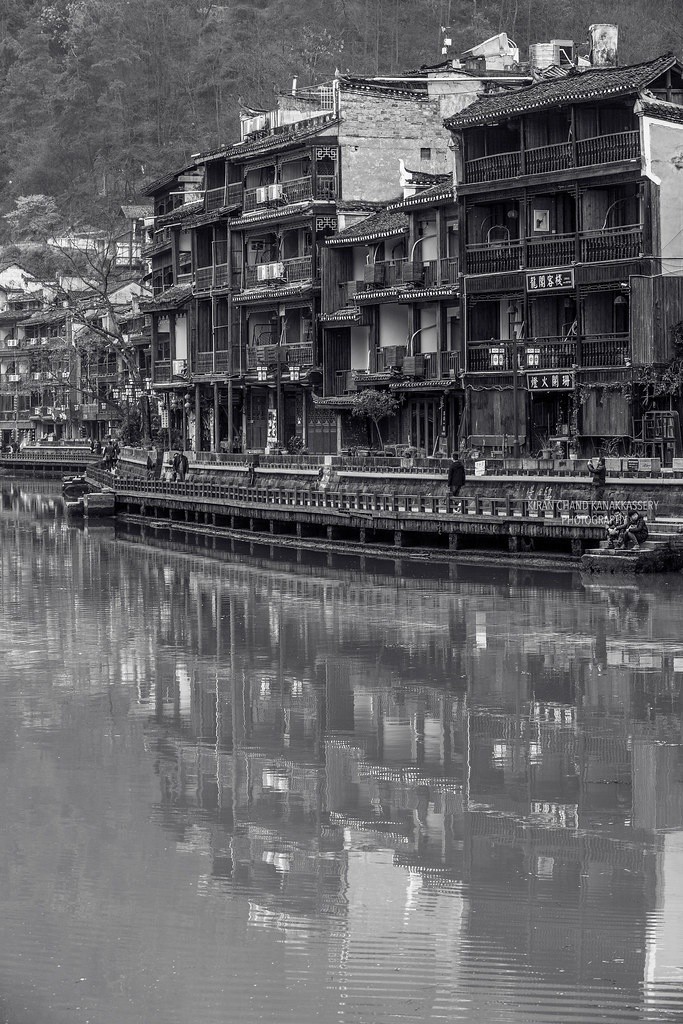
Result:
[8,340,18,346]
[8,375,19,382]
[40,337,50,344]
[47,371,56,379]
[47,408,52,415]
[35,407,40,414]
[35,372,41,381]
[29,338,37,344]
[268,263,285,280]
[255,188,268,203]
[267,185,284,201]
[257,266,270,282]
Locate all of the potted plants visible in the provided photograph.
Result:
[420,448,426,458]
[535,434,551,460]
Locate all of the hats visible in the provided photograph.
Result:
[178,448,183,451]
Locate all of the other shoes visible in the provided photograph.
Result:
[615,545,619,550]
[605,544,614,549]
[631,545,639,550]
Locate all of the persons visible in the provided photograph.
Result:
[604,508,648,550]
[447,453,466,513]
[103,440,120,472]
[11,441,20,453]
[89,439,99,453]
[587,456,606,519]
[0,439,7,453]
[172,449,189,482]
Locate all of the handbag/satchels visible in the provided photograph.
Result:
[106,454,111,462]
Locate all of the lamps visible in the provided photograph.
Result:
[507,201,518,218]
